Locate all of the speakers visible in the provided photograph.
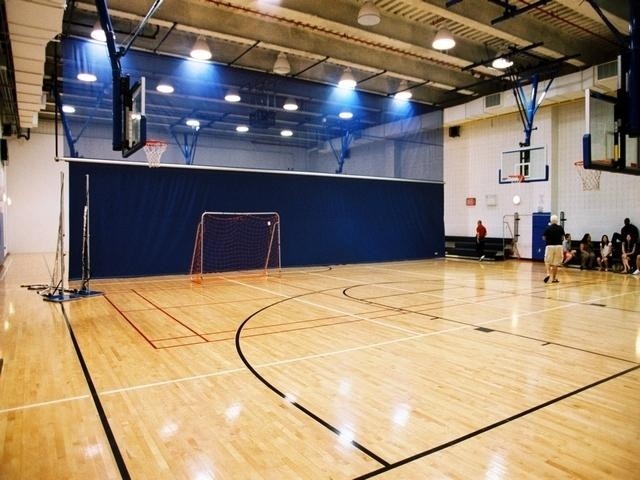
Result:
[449,125,460,137]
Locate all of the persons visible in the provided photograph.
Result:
[476,220,487,261]
[542,215,566,283]
[562,218,640,275]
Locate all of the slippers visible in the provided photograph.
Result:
[552,279,560,283]
[544,276,550,283]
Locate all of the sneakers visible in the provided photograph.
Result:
[580,265,640,275]
[479,255,486,262]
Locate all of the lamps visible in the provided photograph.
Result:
[431,27,455,52]
[272,52,291,75]
[338,107,354,119]
[283,96,299,111]
[338,68,358,88]
[357,2,382,27]
[76,68,98,82]
[490,47,512,70]
[394,79,413,100]
[224,88,242,103]
[189,35,213,62]
[156,77,174,93]
[90,23,114,44]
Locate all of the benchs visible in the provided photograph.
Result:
[566,240,639,274]
[446,240,503,261]
